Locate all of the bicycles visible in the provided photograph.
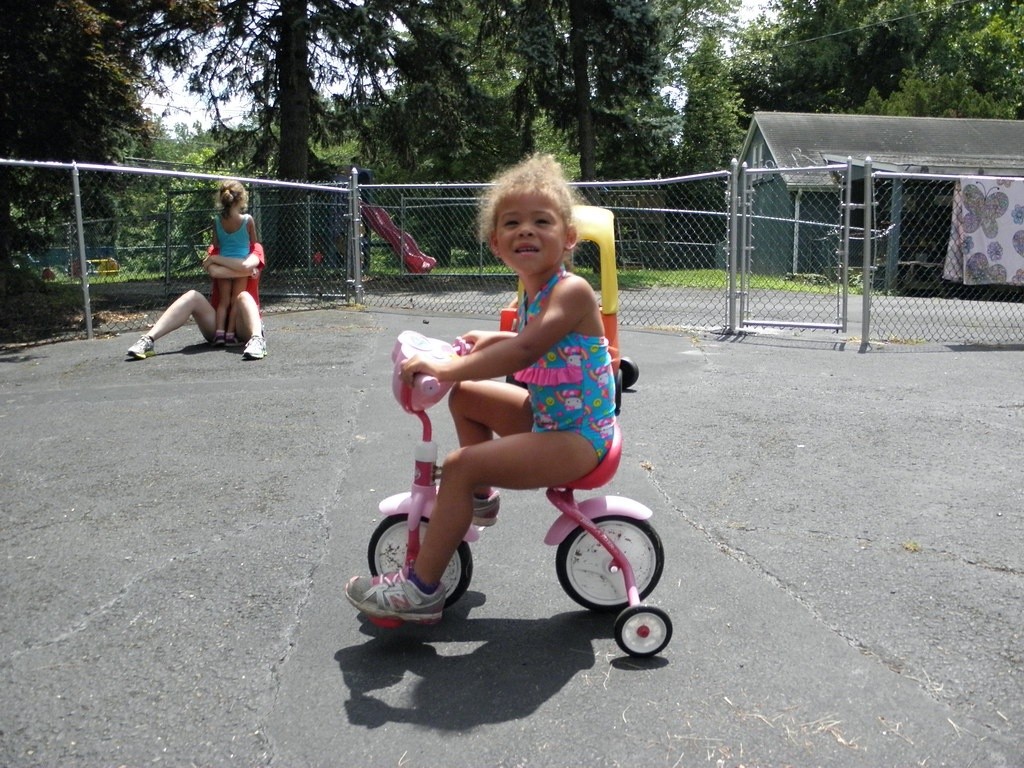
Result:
[367,331,676,658]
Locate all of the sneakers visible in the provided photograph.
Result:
[346,568,446,625]
[242,335,267,358]
[128,334,155,358]
[211,336,243,347]
[473,488,500,526]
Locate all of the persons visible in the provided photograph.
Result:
[125,178,267,360]
[343,155,617,624]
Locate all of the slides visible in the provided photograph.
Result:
[359,198,436,274]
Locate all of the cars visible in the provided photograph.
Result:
[481,202,642,415]
